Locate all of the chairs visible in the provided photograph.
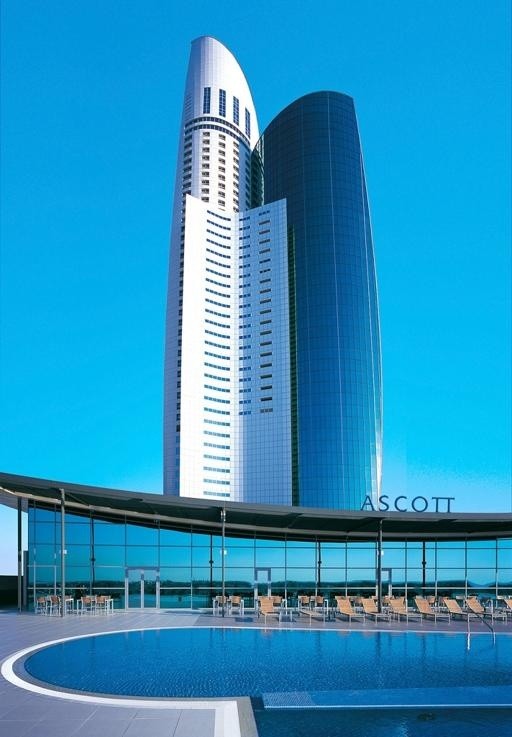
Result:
[34,593,114,616]
[214,596,512,624]
